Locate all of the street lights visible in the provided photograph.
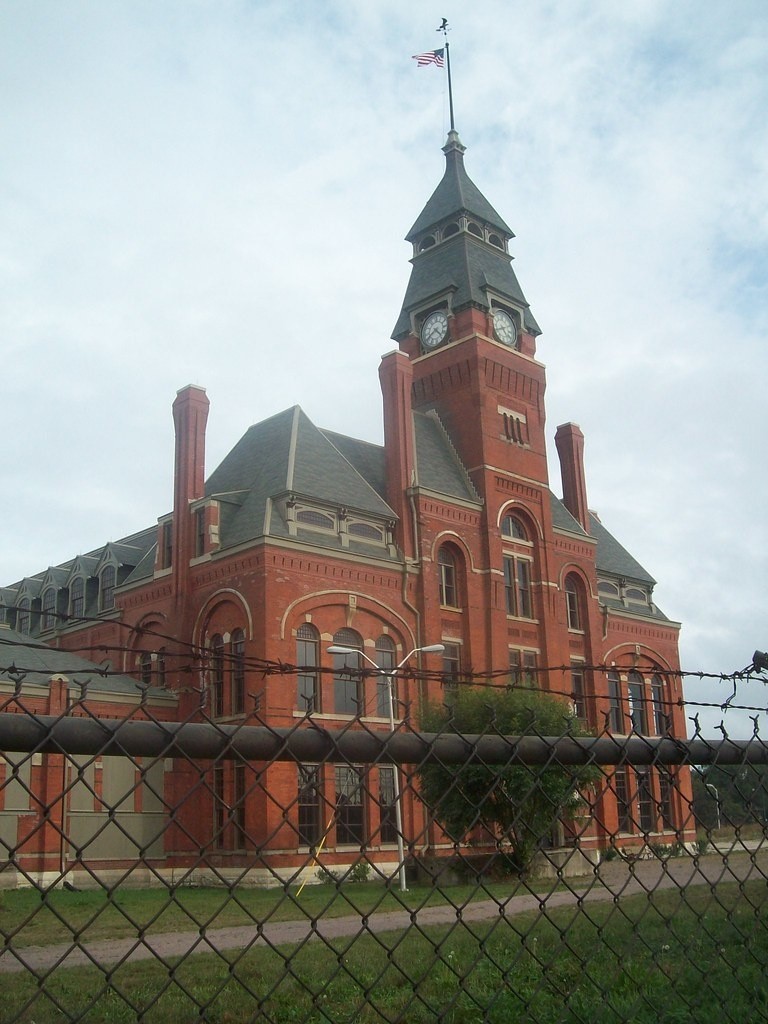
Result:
[327,644,445,892]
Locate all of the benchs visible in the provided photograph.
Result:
[622,845,651,860]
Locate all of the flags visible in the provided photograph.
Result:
[412,48,444,68]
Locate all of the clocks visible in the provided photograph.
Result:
[419,309,451,353]
[492,309,517,347]
